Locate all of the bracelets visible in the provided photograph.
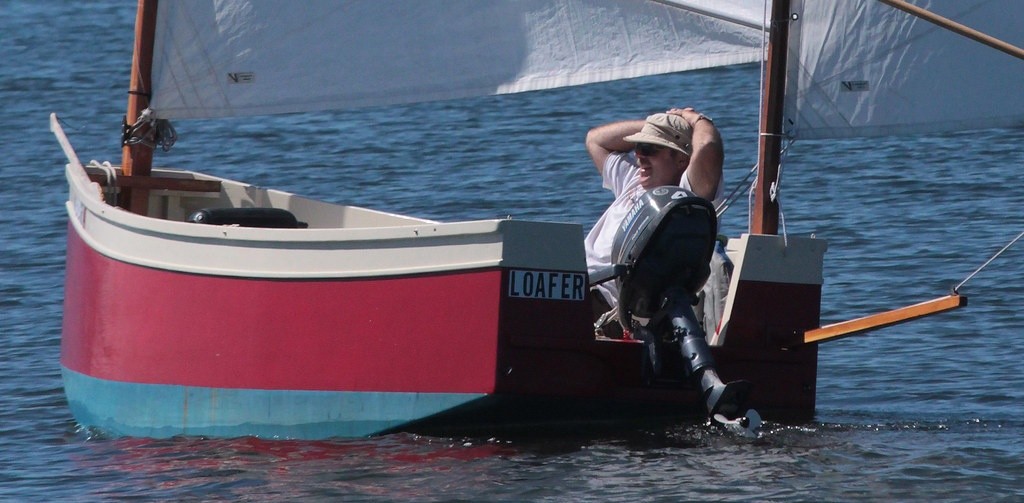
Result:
[696,113,712,122]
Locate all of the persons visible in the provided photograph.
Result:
[583,107,725,338]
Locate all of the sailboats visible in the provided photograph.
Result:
[50,0,1024,448]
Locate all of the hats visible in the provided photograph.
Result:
[623,113,693,156]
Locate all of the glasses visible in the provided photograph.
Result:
[634,142,667,156]
